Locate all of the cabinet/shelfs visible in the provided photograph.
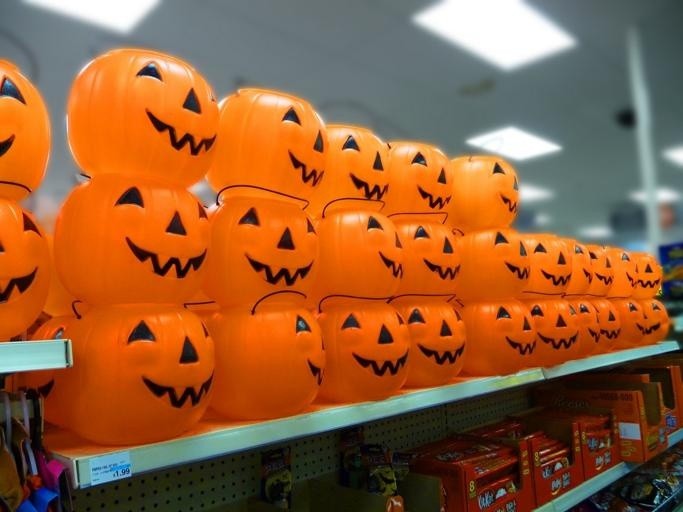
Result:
[0,320,683,512]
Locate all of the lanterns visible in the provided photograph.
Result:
[1,45,330,447]
[307,125,536,402]
[518,233,669,367]
